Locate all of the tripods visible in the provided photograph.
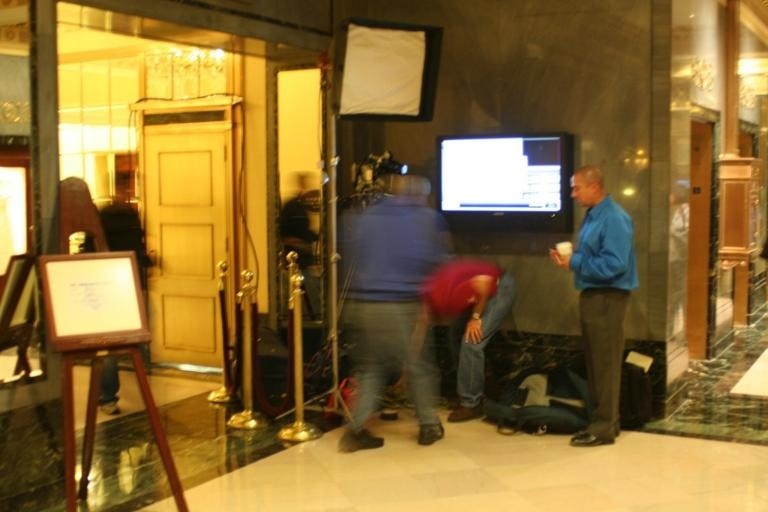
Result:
[274,45,356,428]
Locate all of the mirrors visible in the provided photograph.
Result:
[277,68,322,336]
[0,0,46,389]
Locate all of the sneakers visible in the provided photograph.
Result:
[100,398,120,415]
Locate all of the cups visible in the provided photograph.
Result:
[556,241,572,260]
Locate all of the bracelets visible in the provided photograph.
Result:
[470,312,481,321]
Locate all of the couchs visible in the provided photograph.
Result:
[434,325,666,432]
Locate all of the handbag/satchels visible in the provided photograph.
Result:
[481,360,601,435]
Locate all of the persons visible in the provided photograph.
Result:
[78,169,162,417]
[390,254,519,426]
[548,163,640,449]
[668,178,690,347]
[333,171,444,454]
[278,170,324,322]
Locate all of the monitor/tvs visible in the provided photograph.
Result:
[436,133,575,234]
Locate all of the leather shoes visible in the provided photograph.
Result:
[446,401,483,422]
[340,432,384,453]
[570,423,620,447]
[419,425,444,445]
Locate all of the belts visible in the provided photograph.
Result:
[498,266,512,276]
